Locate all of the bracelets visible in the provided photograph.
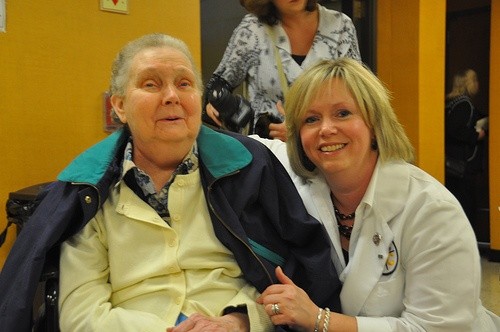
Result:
[311,305,332,332]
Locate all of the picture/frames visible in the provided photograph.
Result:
[99,0,129,14]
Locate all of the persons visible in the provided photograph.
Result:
[56,33,345,332]
[257,54,500,332]
[440,61,488,220]
[203,0,362,141]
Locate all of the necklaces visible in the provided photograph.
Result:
[334,206,355,238]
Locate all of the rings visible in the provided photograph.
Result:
[271,301,282,316]
